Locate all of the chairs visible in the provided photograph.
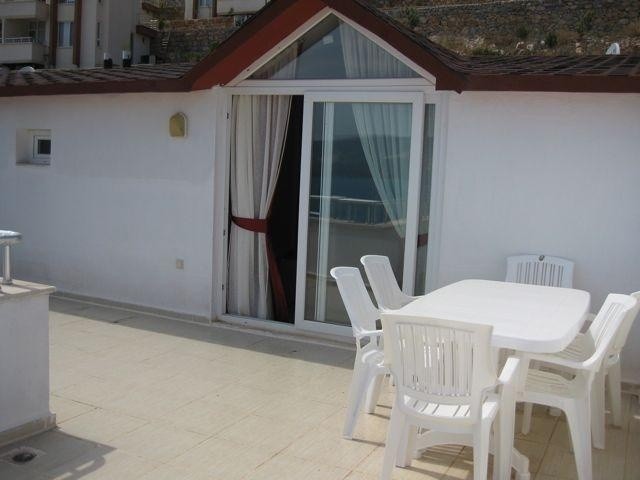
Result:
[330,254,640,479]
